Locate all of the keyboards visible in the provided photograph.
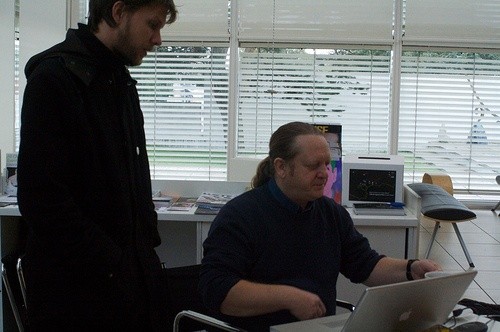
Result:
[352,208,405,216]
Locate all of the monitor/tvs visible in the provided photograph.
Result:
[342,154,404,208]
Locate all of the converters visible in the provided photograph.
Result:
[453,321,488,332]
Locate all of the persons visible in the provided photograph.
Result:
[200,121,442,332]
[16,0,178,331]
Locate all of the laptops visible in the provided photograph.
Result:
[269,270,478,332]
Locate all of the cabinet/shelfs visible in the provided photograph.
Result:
[1,193,418,303]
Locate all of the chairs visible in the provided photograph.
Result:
[160,264,357,332]
[1,251,32,332]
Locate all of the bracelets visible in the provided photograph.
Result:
[406,256,415,280]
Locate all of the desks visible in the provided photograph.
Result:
[269,301,500,332]
[406,182,477,270]
